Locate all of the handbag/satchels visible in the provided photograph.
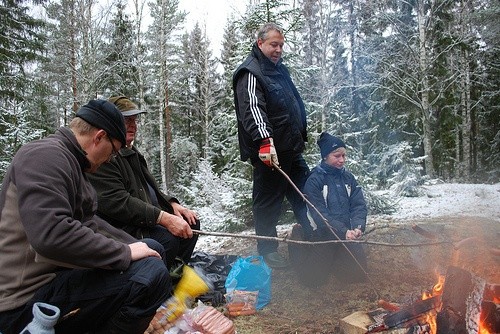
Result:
[224,255,275,309]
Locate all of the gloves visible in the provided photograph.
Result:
[258,137,280,168]
[19,301,60,334]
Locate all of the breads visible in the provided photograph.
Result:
[143,303,183,334]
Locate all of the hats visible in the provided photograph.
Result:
[76,98,127,149]
[317,132,347,158]
[108,95,149,117]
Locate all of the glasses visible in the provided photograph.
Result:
[107,138,121,162]
[123,117,141,126]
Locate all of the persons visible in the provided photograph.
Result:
[0,100,171,334]
[233,23,311,268]
[301,132,367,282]
[88,97,200,270]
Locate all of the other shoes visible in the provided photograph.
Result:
[288,224,304,251]
[262,251,291,268]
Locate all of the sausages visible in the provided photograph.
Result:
[489,246,500,256]
[378,299,401,311]
[224,302,257,318]
[192,306,237,334]
[410,224,441,241]
[453,237,475,250]
